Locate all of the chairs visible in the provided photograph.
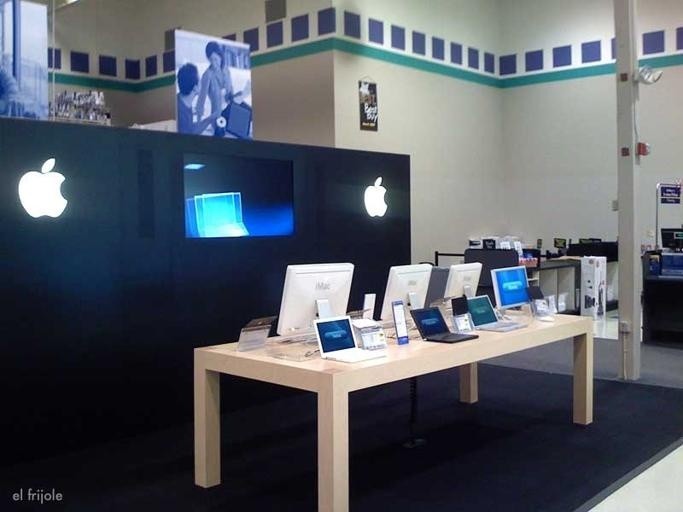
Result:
[464,249,519,307]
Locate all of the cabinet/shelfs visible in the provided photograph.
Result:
[526,259,581,315]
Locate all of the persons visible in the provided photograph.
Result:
[177,41,251,135]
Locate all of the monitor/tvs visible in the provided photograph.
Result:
[378,263,432,320]
[443,262,483,300]
[490,264,532,318]
[276,262,354,335]
[661,228,683,253]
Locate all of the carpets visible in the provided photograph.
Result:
[2,359,683,512]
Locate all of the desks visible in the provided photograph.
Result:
[642,249,683,350]
[194,308,594,512]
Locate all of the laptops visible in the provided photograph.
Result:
[313,315,386,363]
[466,294,527,332]
[410,307,478,343]
[220,103,250,138]
